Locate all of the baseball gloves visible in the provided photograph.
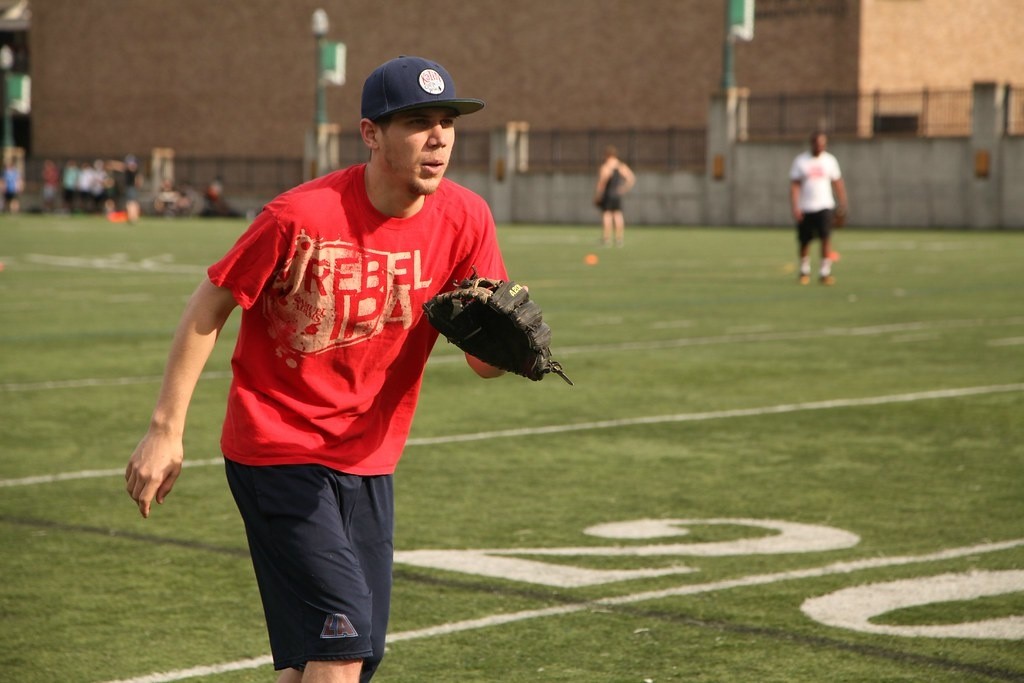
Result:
[422,264,575,386]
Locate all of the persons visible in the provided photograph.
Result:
[6,152,226,226]
[594,145,636,249]
[789,131,847,286]
[124,57,552,682]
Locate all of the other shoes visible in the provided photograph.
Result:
[821,275,836,286]
[799,271,811,286]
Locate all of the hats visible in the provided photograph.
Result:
[360,54,486,123]
